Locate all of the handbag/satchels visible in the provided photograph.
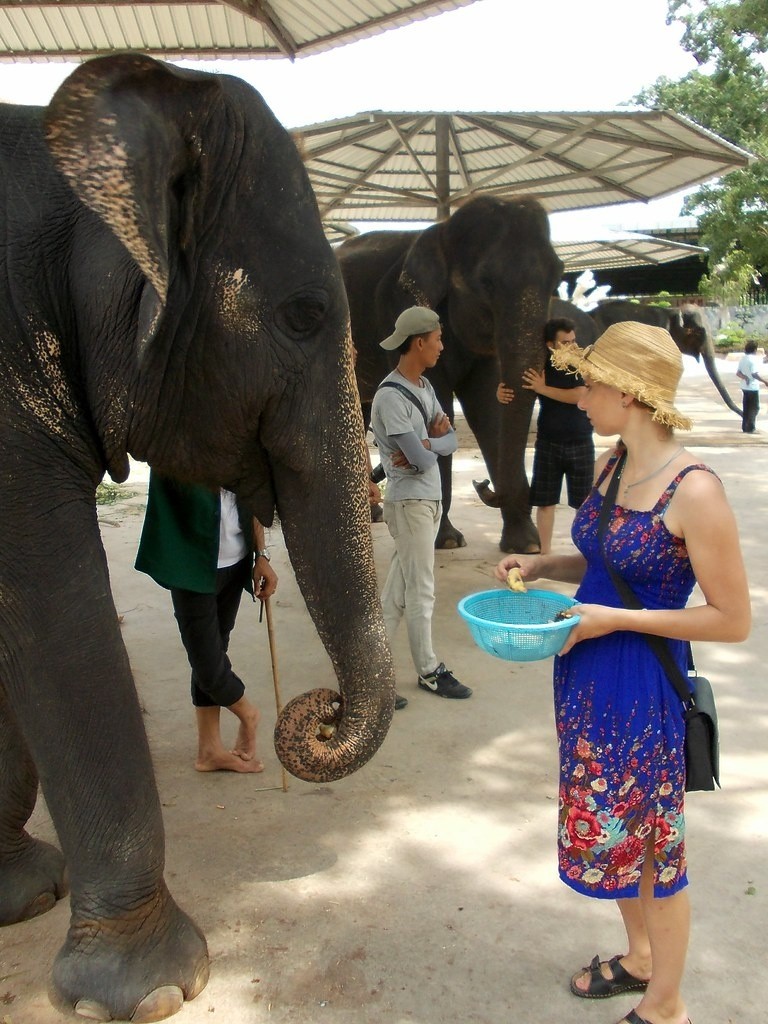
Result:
[683,669,720,794]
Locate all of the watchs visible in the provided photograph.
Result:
[255,549,271,562]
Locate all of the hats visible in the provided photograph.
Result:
[379,305,440,350]
[549,320,695,431]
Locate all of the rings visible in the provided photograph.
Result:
[273,588,276,591]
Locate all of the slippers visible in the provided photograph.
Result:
[616,1009,693,1024]
[570,954,650,999]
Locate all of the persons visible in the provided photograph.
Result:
[495,318,595,555]
[371,305,471,708]
[736,340,768,434]
[134,465,278,774]
[494,321,752,1024]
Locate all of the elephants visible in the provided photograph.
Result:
[329,193,564,557]
[0,49,397,1024]
[584,300,744,419]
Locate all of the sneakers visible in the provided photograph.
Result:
[417,662,473,699]
[395,694,408,710]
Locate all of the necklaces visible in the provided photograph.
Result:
[618,442,684,498]
[396,366,421,389]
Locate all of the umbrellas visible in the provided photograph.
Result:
[284,110,754,223]
[0,0,480,63]
[537,230,712,274]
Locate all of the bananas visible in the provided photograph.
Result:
[506,567,527,593]
[318,723,337,738]
